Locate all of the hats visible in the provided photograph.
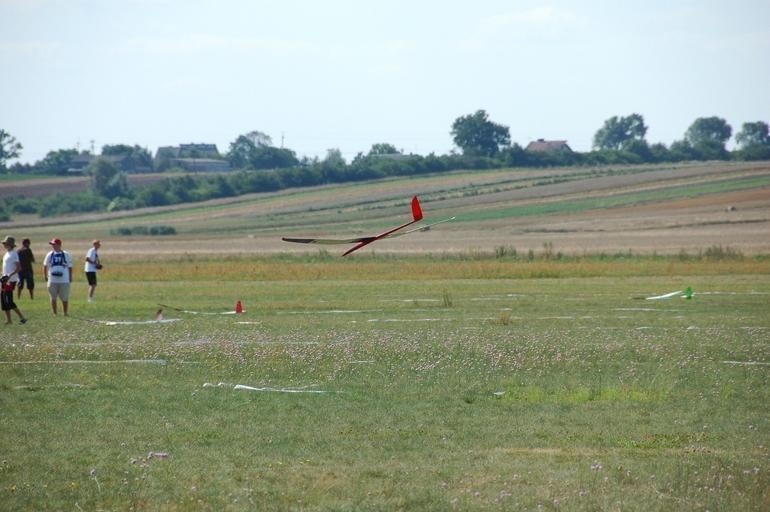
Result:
[1,237,17,247]
[49,238,62,246]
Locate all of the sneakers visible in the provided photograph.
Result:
[19,319,28,325]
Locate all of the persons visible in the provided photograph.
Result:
[16,238,37,300]
[42,238,74,318]
[84,239,104,303]
[0,235,28,326]
